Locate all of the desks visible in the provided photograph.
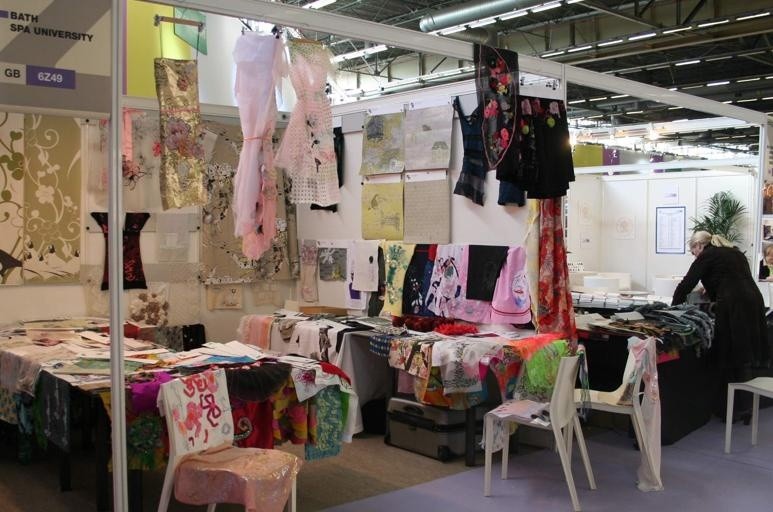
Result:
[576,325,718,451]
[244,306,571,466]
[0,315,337,512]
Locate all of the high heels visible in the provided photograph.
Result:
[732,409,750,425]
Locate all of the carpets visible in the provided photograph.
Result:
[316,403,773,512]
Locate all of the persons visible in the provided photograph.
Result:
[671,230,770,426]
[764,184,770,189]
[762,187,769,214]
[766,184,773,214]
[759,242,773,280]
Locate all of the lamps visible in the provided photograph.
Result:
[273,0,772,127]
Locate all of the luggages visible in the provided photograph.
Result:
[383,397,488,462]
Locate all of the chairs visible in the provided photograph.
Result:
[482,336,664,512]
[724,376,773,455]
[155,368,300,512]
